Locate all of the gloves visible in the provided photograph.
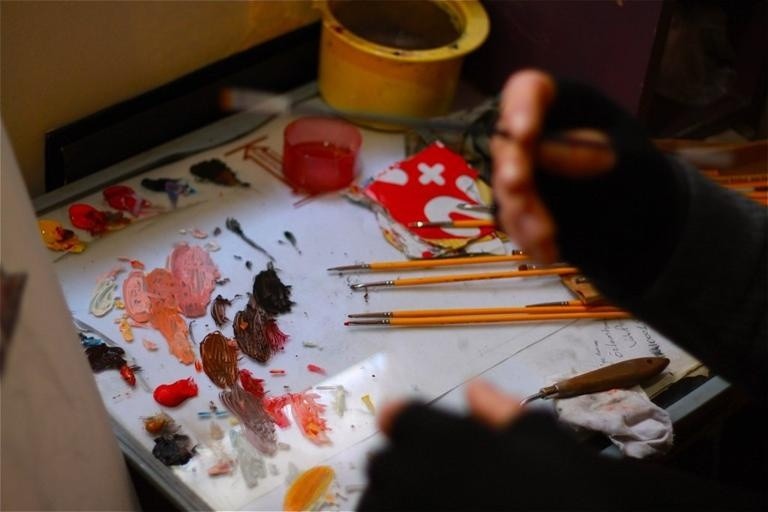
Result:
[513,69,688,311]
[351,399,669,511]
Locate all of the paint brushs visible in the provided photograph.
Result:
[456,203,496,210]
[344,300,632,326]
[328,255,580,289]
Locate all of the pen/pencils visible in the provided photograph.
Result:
[412,220,496,227]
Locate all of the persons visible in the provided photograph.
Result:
[351,65,766,510]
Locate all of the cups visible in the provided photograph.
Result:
[316,2,490,132]
[281,115,362,196]
